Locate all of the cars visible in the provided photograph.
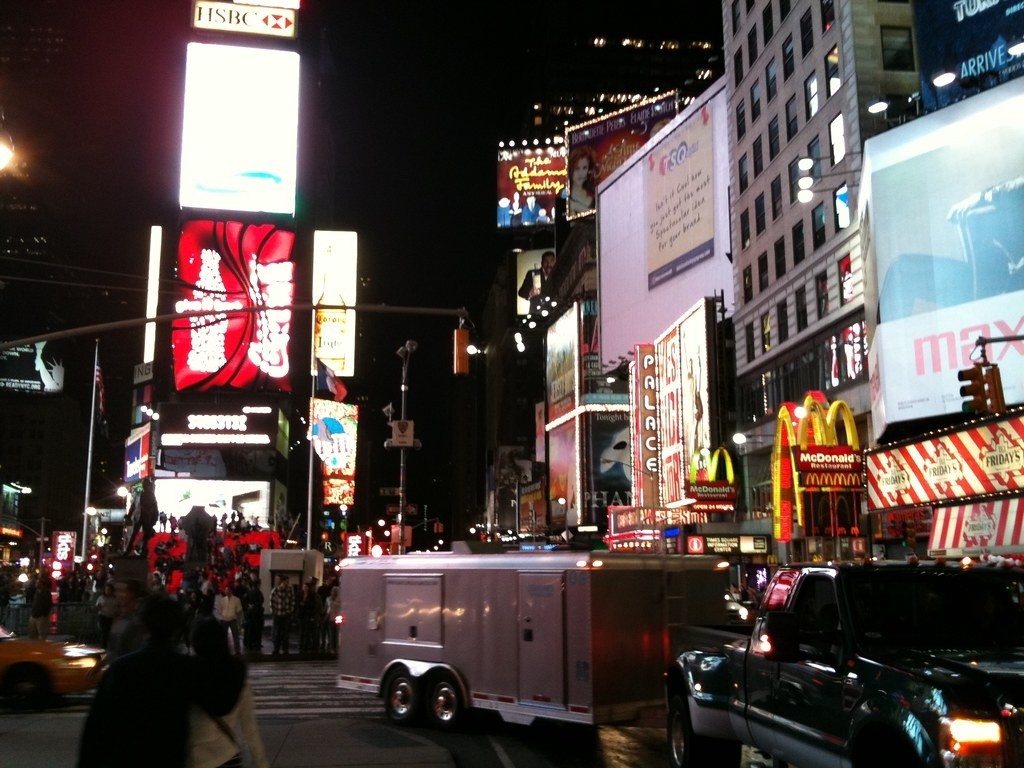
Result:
[0,624,111,714]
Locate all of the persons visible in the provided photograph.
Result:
[518,251,558,316]
[0,509,339,656]
[878,170,1024,324]
[730,578,769,617]
[181,617,269,768]
[499,192,557,227]
[73,601,193,768]
[568,146,596,216]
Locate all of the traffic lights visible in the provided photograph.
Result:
[956,363,990,419]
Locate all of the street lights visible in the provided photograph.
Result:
[395,341,419,554]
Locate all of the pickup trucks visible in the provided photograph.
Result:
[652,554,1024,764]
